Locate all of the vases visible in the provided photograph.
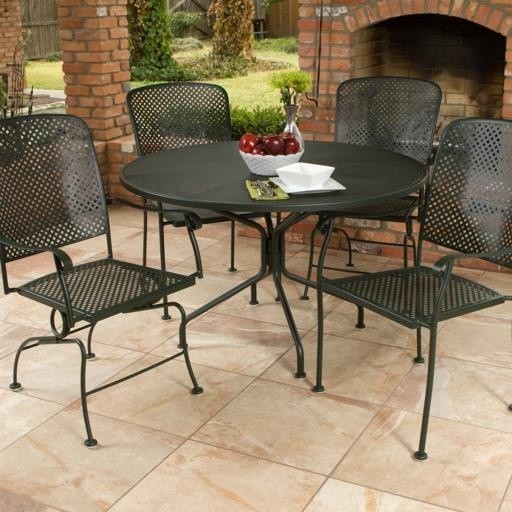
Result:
[281,104,303,147]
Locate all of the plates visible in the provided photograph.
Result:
[268,178,345,197]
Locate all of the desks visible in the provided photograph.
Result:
[119,138,430,381]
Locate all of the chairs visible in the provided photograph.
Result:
[301,76,443,302]
[124,81,287,320]
[0,112,202,446]
[319,115,512,462]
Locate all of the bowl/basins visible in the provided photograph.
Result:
[276,162,335,187]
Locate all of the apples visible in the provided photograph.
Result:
[239,132,299,155]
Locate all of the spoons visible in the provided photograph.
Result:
[251,181,277,197]
